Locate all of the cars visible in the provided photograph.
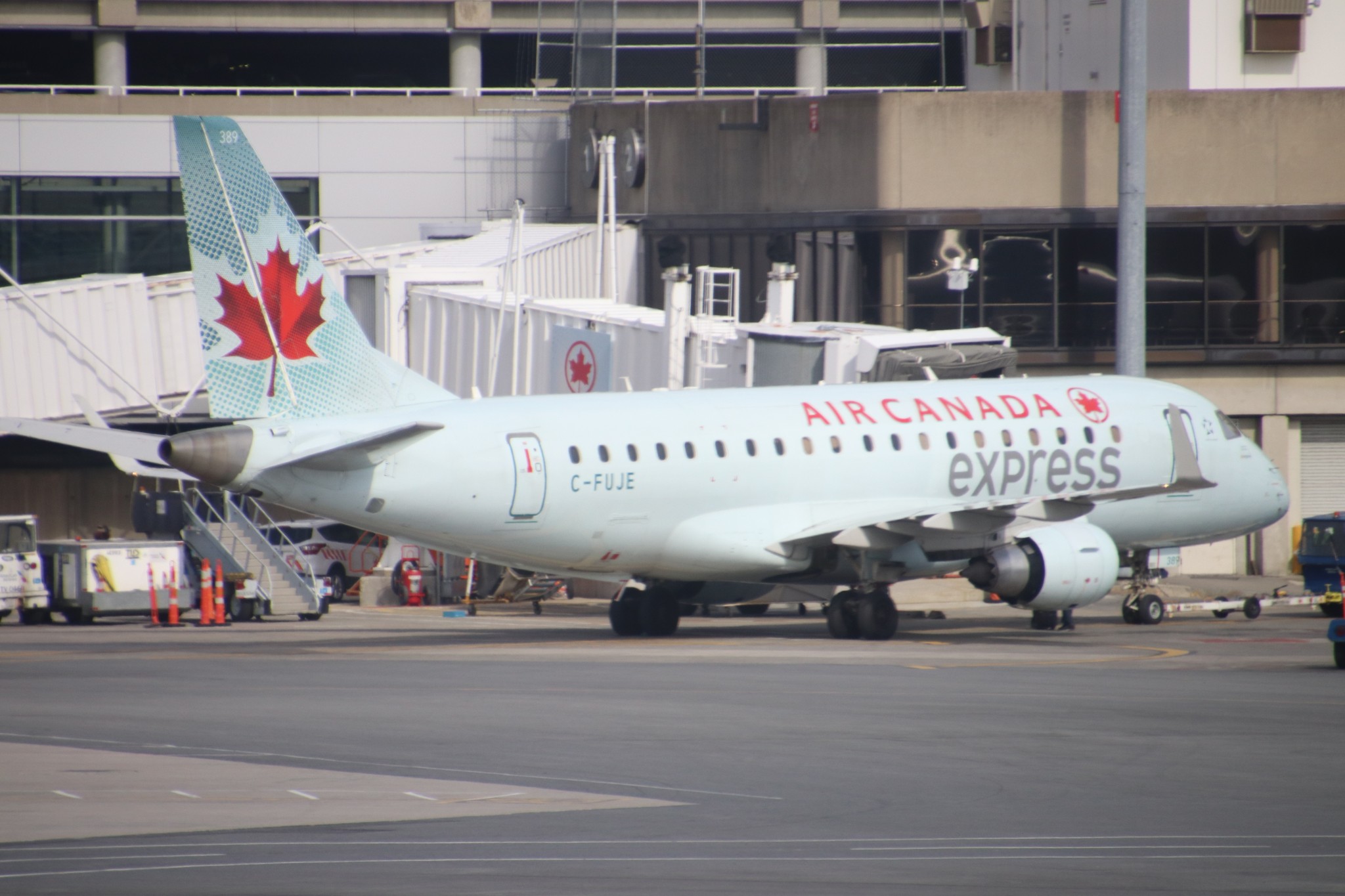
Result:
[1297,510,1345,668]
[259,518,387,602]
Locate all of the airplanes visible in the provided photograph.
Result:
[158,112,1293,642]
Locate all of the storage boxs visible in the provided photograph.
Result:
[0,221,746,420]
